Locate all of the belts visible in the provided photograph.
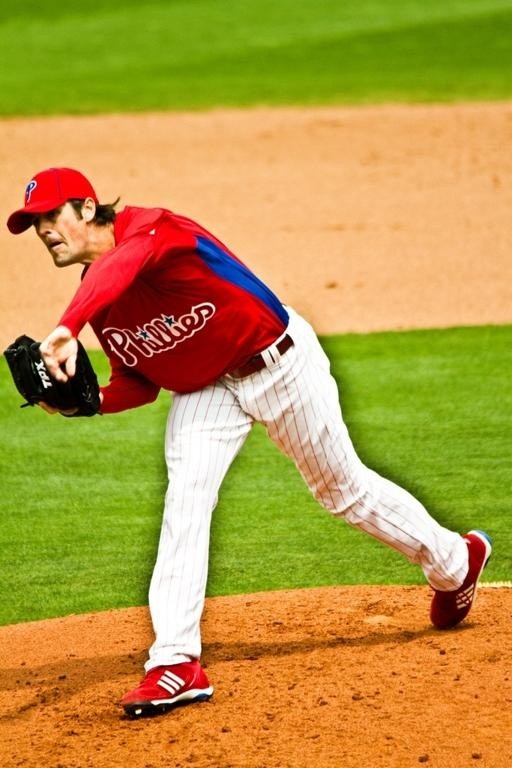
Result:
[230,335,294,378]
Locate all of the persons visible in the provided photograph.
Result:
[3,167,493,716]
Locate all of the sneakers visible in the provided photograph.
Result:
[430,530,492,629]
[121,658,215,715]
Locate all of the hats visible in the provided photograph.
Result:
[7,168,98,235]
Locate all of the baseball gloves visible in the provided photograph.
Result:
[4,335,100,417]
